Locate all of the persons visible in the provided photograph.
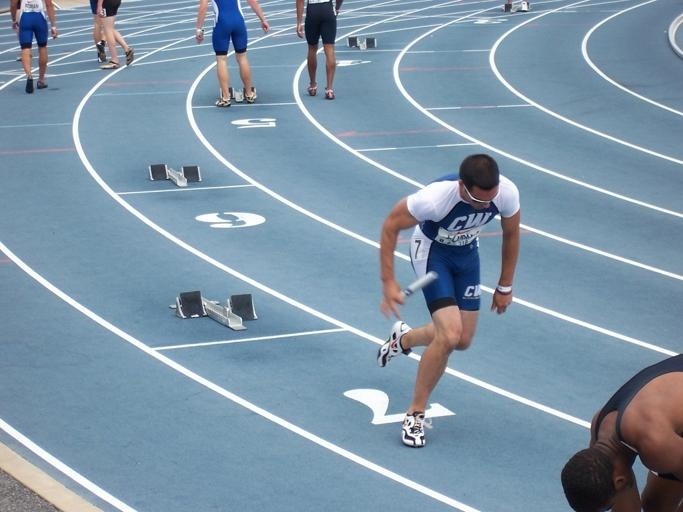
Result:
[561,354,683,512]
[377,154,520,448]
[97,0,133,69]
[296,0,343,100]
[11,1,57,93]
[194,0,270,106]
[90,0,106,64]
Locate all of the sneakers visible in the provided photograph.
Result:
[215,97,231,107]
[377,321,412,367]
[245,91,256,103]
[308,87,317,96]
[401,411,433,447]
[325,88,334,99]
[18,39,133,92]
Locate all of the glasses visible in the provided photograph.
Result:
[463,184,500,205]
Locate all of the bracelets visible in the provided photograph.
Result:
[195,28,202,33]
[494,285,513,296]
[50,26,56,30]
